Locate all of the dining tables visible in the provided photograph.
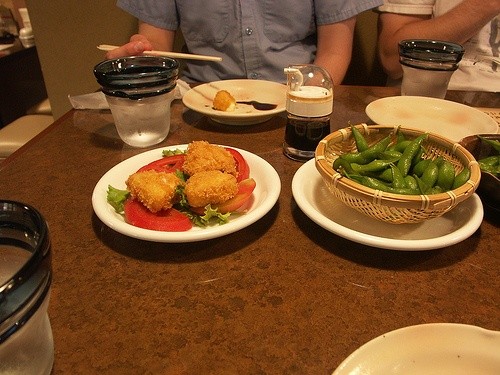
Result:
[0,81,500,375]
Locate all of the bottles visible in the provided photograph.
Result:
[283,64,333,163]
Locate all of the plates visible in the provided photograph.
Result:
[182,79,287,125]
[91,144,281,243]
[292,157,484,251]
[365,96,499,143]
[331,323,500,375]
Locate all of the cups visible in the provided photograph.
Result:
[398,39,466,99]
[0,200,54,375]
[93,56,180,148]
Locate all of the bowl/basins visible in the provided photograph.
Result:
[458,134,500,186]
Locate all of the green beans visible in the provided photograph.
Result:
[473,135,500,180]
[332,120,471,195]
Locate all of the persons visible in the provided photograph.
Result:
[371,0,500,91]
[105,0,385,86]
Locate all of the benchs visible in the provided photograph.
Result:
[0,93,53,158]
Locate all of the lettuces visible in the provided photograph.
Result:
[106,149,231,229]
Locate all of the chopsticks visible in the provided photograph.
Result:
[96,44,223,64]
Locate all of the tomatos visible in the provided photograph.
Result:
[124,196,193,232]
[224,148,249,183]
[136,154,186,175]
[189,178,256,215]
